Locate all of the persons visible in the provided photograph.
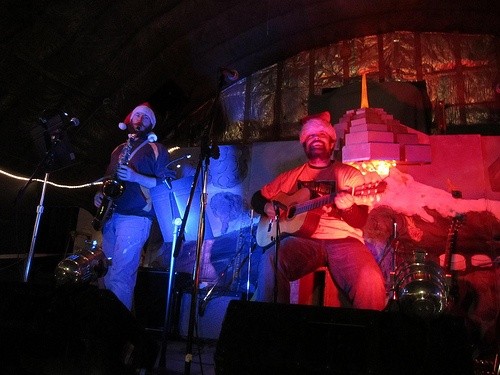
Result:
[94,101,175,311]
[251,118,389,310]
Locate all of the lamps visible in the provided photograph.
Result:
[394,258,449,322]
[56,243,109,286]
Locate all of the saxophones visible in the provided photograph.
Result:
[92,125,140,230]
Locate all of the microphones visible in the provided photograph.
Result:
[64,117,80,129]
[218,67,239,81]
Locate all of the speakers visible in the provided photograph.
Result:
[213,299,477,375]
[0,277,160,375]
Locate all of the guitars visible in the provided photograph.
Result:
[441,213,465,300]
[256,180,388,248]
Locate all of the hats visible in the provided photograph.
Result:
[118,102,156,130]
[298,111,337,145]
